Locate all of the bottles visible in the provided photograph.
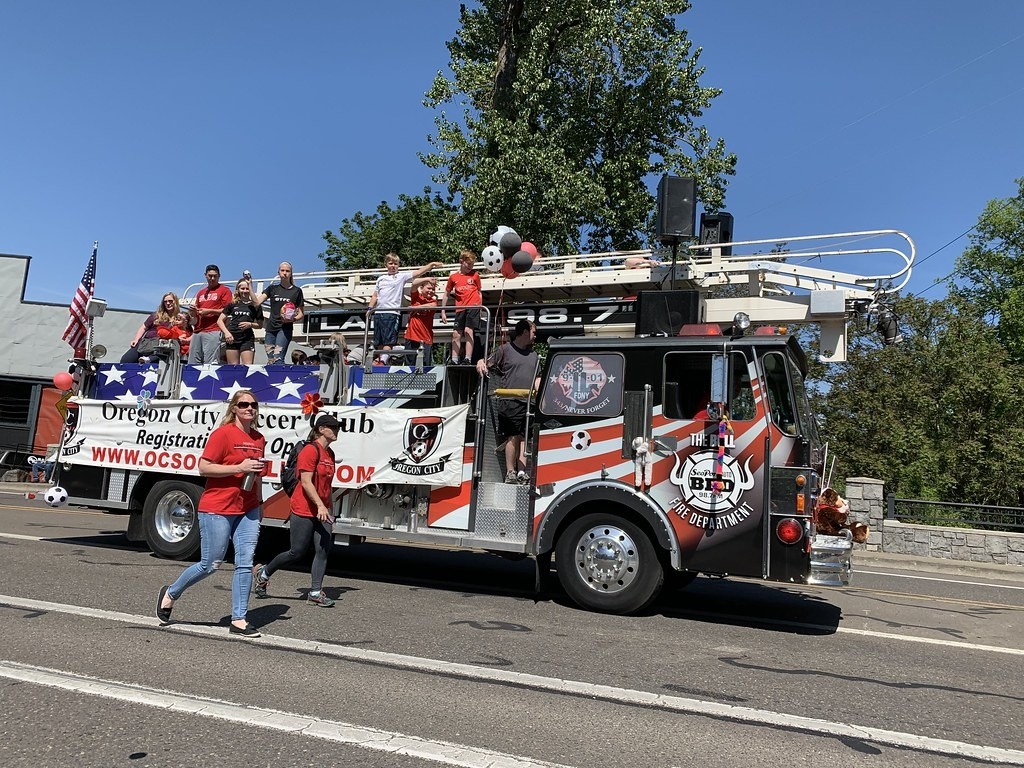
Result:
[241,460,261,491]
[408,510,418,532]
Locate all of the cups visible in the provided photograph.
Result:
[384,516,391,528]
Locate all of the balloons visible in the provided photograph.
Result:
[481,224,538,280]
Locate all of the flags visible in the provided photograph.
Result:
[60,247,99,354]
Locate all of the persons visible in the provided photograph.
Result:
[291,332,351,366]
[30,462,54,483]
[119,291,194,364]
[187,264,233,365]
[155,389,268,638]
[244,261,304,365]
[365,252,443,367]
[475,319,543,485]
[252,414,347,607]
[216,277,264,365]
[441,248,483,365]
[402,277,439,367]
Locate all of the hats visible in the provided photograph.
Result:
[316,415,347,428]
[156,326,179,339]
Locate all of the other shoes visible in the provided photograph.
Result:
[40,480,47,483]
[31,479,39,483]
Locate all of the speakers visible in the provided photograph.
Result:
[635,290,707,337]
[698,212,734,259]
[657,176,696,243]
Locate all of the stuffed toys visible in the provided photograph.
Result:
[815,487,869,544]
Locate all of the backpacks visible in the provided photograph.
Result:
[281,440,336,498]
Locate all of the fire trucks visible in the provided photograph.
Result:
[24,177,919,614]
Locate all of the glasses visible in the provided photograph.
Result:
[324,426,340,432]
[164,300,174,303]
[204,288,210,300]
[235,402,259,409]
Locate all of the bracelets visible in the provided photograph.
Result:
[367,307,373,310]
[250,322,254,328]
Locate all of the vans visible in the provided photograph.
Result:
[0,449,45,478]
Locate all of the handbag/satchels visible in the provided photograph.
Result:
[137,337,161,355]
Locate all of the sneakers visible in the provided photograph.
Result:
[156,586,174,624]
[505,469,530,485]
[447,359,460,365]
[306,591,335,606]
[254,564,269,598]
[230,622,261,637]
[462,358,471,365]
